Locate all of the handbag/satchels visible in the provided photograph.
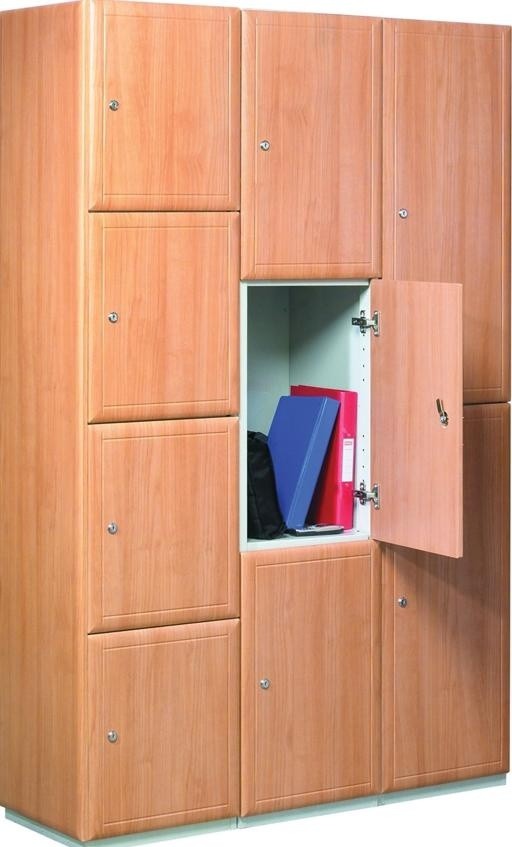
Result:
[247,431,287,540]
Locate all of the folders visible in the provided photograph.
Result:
[268,384,357,529]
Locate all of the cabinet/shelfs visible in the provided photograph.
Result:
[0,1,512,847]
